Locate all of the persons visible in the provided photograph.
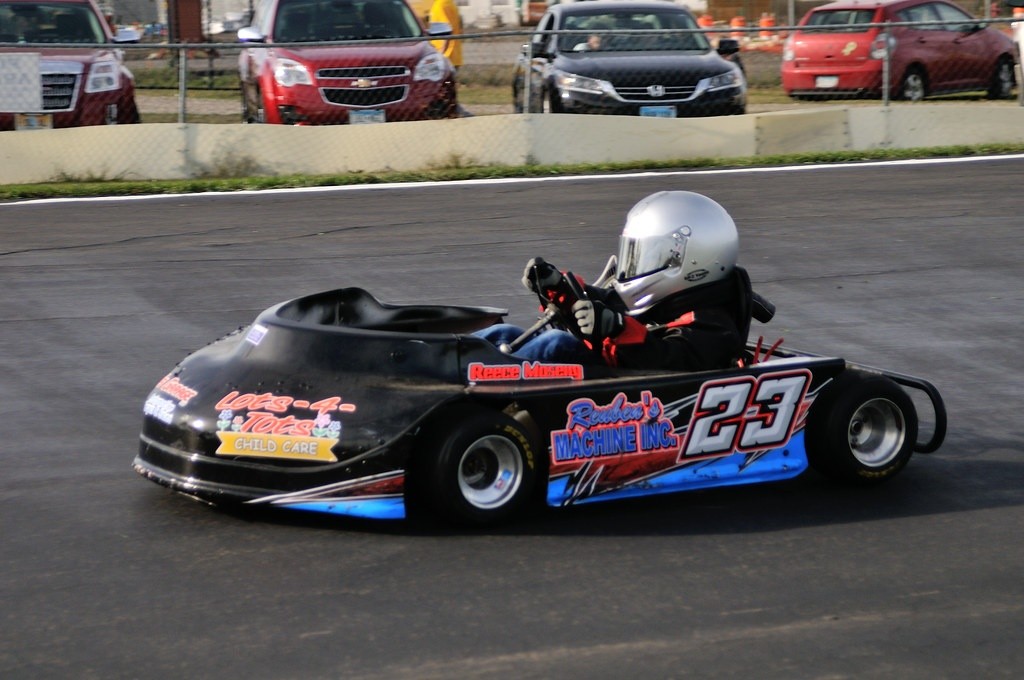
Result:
[697,11,788,46]
[470,191,753,381]
[10,4,43,43]
[574,28,609,51]
[428,0,473,117]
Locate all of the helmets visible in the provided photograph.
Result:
[613,191,738,313]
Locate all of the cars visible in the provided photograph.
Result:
[781,1,1021,100]
[1,1,142,132]
[510,2,748,117]
[237,1,458,125]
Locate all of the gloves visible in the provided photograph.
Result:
[523,256,565,299]
[572,300,623,340]
